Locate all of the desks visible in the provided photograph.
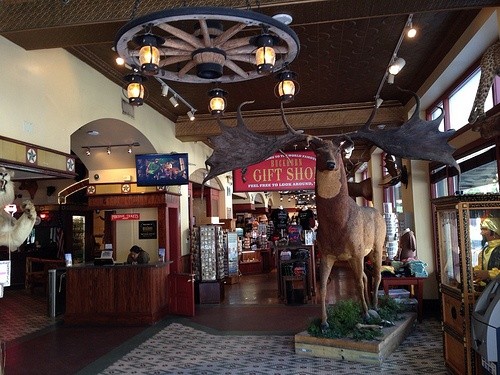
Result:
[381,275,428,306]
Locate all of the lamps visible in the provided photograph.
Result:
[161,84,169,97]
[86,147,91,157]
[121,72,146,107]
[132,24,166,76]
[168,93,179,108]
[274,64,301,104]
[388,55,406,75]
[404,22,417,38]
[207,83,227,116]
[106,147,111,155]
[280,192,317,210]
[187,109,195,121]
[127,146,132,154]
[248,33,282,77]
[388,73,395,84]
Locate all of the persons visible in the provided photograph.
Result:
[270,205,289,231]
[298,205,315,230]
[400,228,417,260]
[124,246,150,265]
[472,216,500,292]
[268,231,282,241]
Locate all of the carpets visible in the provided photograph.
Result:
[74,316,459,375]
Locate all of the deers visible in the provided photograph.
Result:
[202,90,461,328]
